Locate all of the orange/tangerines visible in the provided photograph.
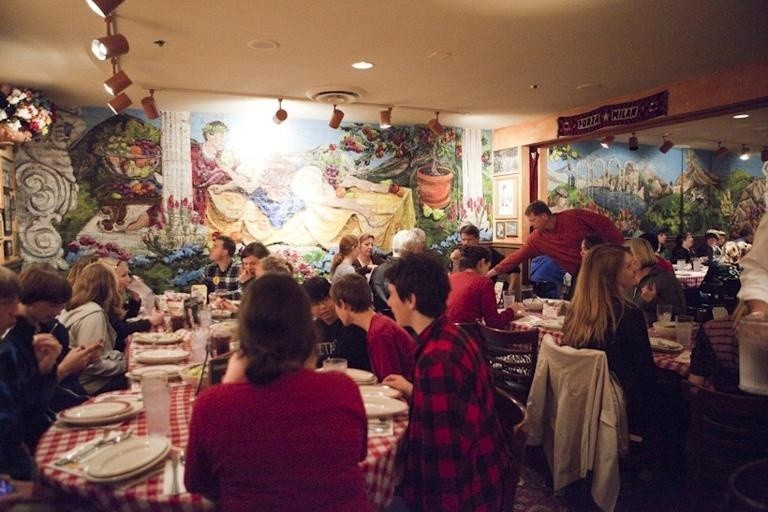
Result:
[397,188,405,197]
[130,146,142,154]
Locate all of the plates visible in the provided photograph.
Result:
[57,395,147,425]
[125,330,193,385]
[81,432,172,487]
[649,337,684,354]
[316,366,412,420]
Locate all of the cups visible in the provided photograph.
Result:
[656,302,694,346]
[676,258,703,272]
[737,311,767,399]
[502,289,516,310]
[322,357,346,373]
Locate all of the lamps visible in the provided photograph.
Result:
[270,101,447,140]
[597,133,614,148]
[139,91,160,123]
[627,132,768,165]
[82,0,135,116]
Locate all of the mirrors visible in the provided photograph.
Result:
[528,98,768,243]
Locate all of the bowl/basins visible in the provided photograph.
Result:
[524,298,554,313]
[651,321,698,339]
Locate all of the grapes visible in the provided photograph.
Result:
[324,165,340,185]
[112,181,134,199]
[136,138,159,155]
[107,133,128,153]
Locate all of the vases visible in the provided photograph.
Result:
[417,165,453,209]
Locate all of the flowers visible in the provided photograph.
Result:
[445,194,492,228]
[1,83,56,147]
[143,194,207,267]
[66,234,135,272]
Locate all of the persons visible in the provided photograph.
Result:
[2,228,507,512]
[442,198,768,512]
[210,135,392,227]
[128,120,238,232]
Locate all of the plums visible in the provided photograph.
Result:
[481,152,489,162]
[329,127,433,158]
[390,183,399,194]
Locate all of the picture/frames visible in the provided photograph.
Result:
[492,145,520,240]
